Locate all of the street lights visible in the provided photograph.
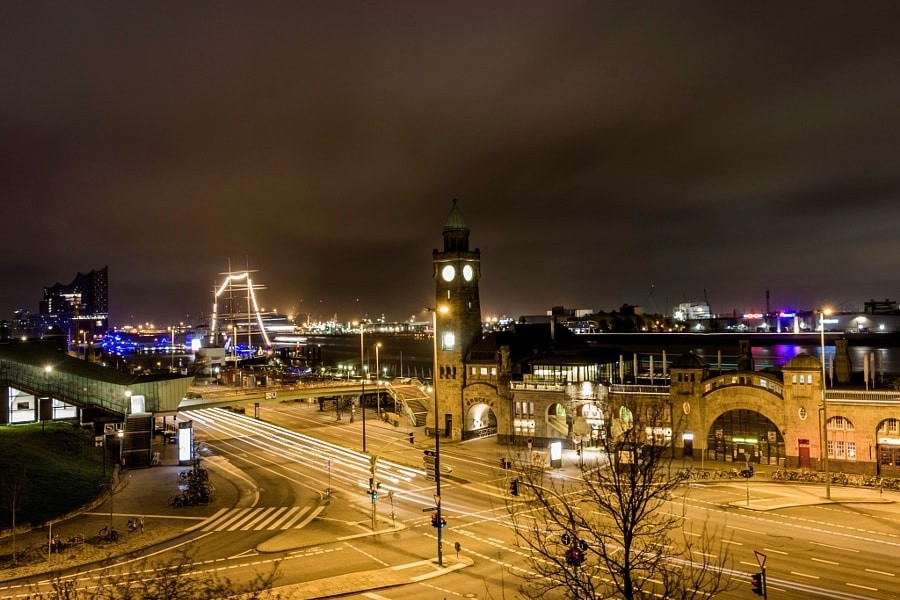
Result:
[423,309,442,566]
[352,321,365,453]
[819,305,834,498]
[376,343,382,417]
[118,429,124,473]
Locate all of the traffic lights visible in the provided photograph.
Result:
[751,573,763,597]
[372,491,377,499]
[566,548,585,566]
[432,514,446,528]
[510,479,518,496]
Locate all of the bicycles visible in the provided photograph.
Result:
[771,466,900,490]
[41,517,144,554]
[676,468,752,480]
[180,468,209,484]
[169,484,210,508]
